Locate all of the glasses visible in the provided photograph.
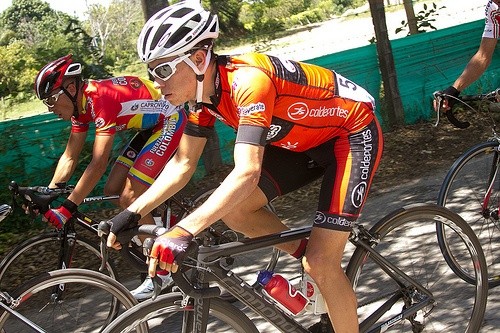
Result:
[146,44,208,82]
[43,81,75,108]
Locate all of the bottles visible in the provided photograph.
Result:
[257,269,309,317]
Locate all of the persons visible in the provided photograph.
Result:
[433,0,500,113]
[20,54,188,300]
[100,0,383,333]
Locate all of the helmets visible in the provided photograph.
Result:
[33,53,82,101]
[136,0,219,62]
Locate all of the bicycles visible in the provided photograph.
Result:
[97,156,489,333]
[0,180,281,333]
[431,88,500,288]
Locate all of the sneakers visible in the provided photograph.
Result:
[130,270,174,299]
